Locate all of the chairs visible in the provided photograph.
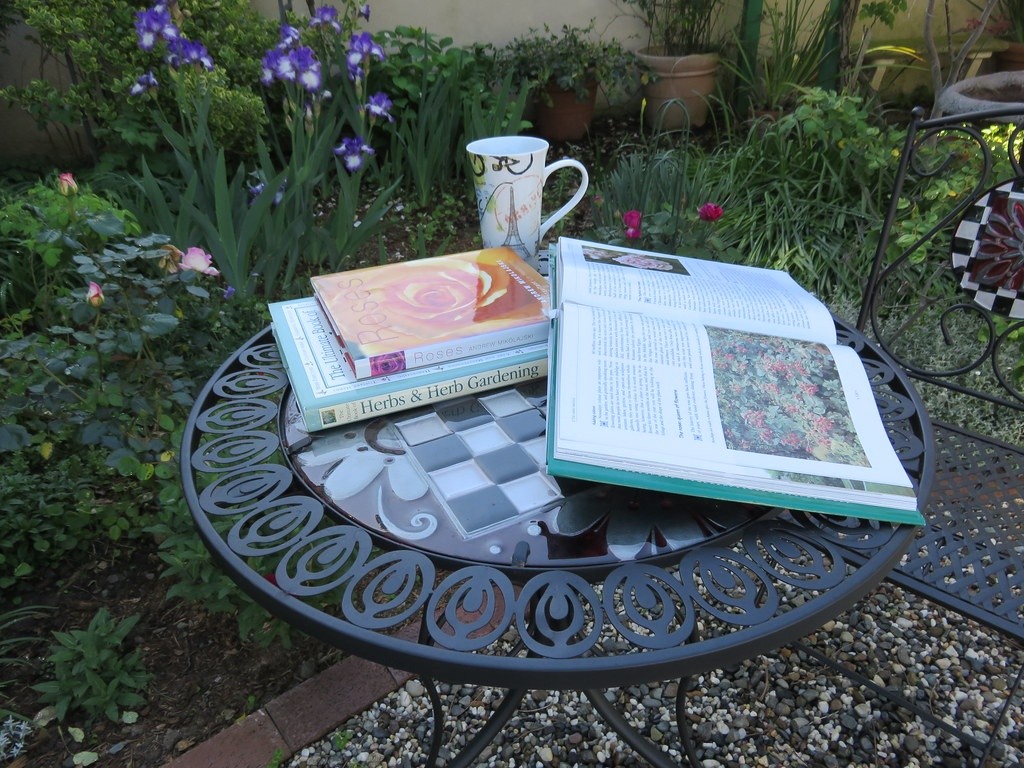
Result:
[726,106,1024,768]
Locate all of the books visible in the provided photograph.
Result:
[545,235,926,526]
[268,245,552,434]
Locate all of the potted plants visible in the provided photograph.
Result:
[622,0,725,130]
[487,15,640,140]
[706,0,842,135]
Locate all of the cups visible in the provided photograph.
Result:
[466,134,591,273]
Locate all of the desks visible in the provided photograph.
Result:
[180,313,936,768]
